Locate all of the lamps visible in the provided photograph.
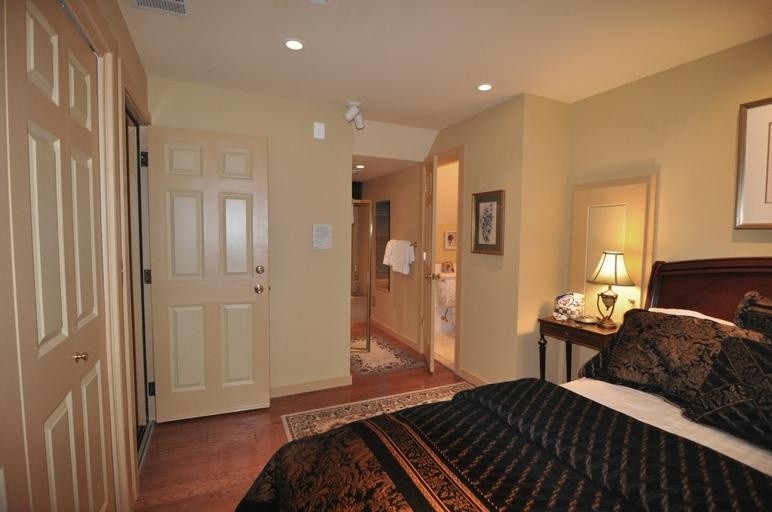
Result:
[587,250,636,330]
[343,100,365,130]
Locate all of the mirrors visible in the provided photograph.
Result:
[375,200,390,293]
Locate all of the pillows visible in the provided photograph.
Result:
[683,331,772,452]
[578,307,737,411]
[732,291,772,337]
[647,308,735,327]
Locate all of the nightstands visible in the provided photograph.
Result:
[538,312,623,381]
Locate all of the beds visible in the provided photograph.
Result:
[235,257,772,512]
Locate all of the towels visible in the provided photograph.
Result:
[383,239,415,276]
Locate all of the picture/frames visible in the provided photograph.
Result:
[444,231,457,250]
[470,189,505,256]
[442,262,454,273]
[568,176,657,325]
[733,97,772,230]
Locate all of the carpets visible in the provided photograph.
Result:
[350,325,426,380]
[280,381,477,444]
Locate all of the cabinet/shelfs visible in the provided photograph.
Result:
[0,0,134,511]
